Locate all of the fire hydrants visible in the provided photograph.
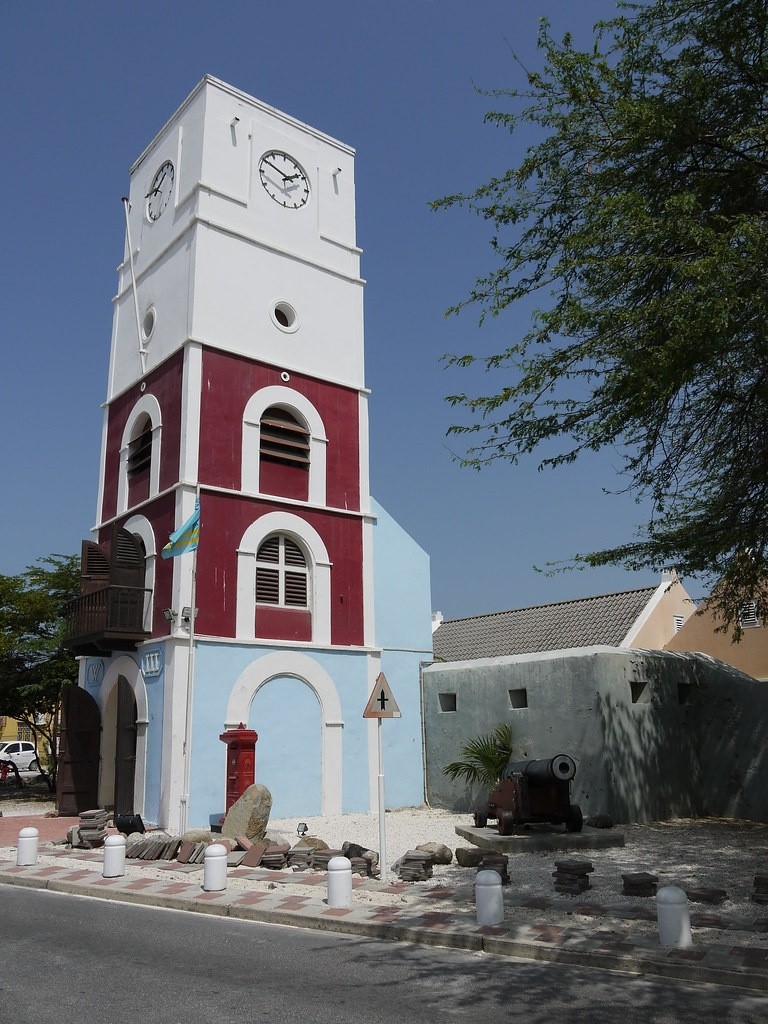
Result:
[0,764,10,780]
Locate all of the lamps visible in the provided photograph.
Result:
[182,607,199,622]
[162,608,178,621]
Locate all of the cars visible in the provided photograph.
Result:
[0,741,39,771]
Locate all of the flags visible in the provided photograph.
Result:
[161,498,201,558]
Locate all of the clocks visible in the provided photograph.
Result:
[255,149,311,210]
[143,158,175,223]
